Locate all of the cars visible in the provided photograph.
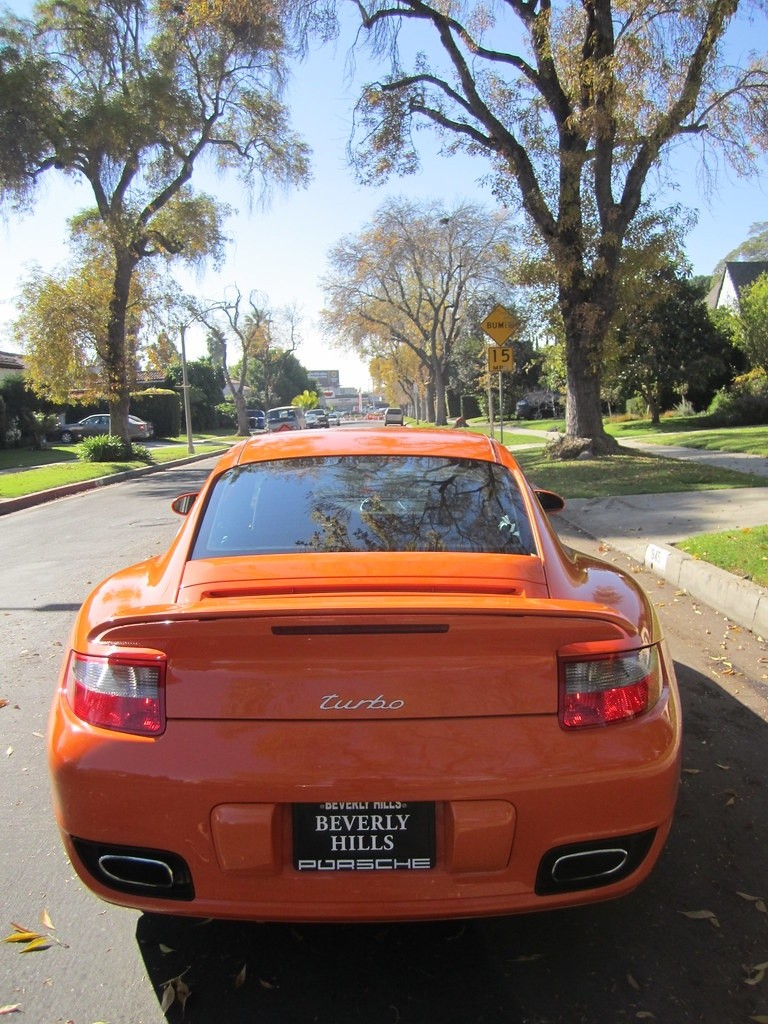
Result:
[59,414,154,444]
[234,410,265,429]
[329,414,340,426]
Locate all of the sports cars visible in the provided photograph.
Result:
[49,426,683,924]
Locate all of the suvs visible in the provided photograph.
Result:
[305,409,329,428]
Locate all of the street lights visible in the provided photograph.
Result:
[178,305,237,454]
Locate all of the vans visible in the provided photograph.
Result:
[267,405,307,431]
[384,408,403,426]
